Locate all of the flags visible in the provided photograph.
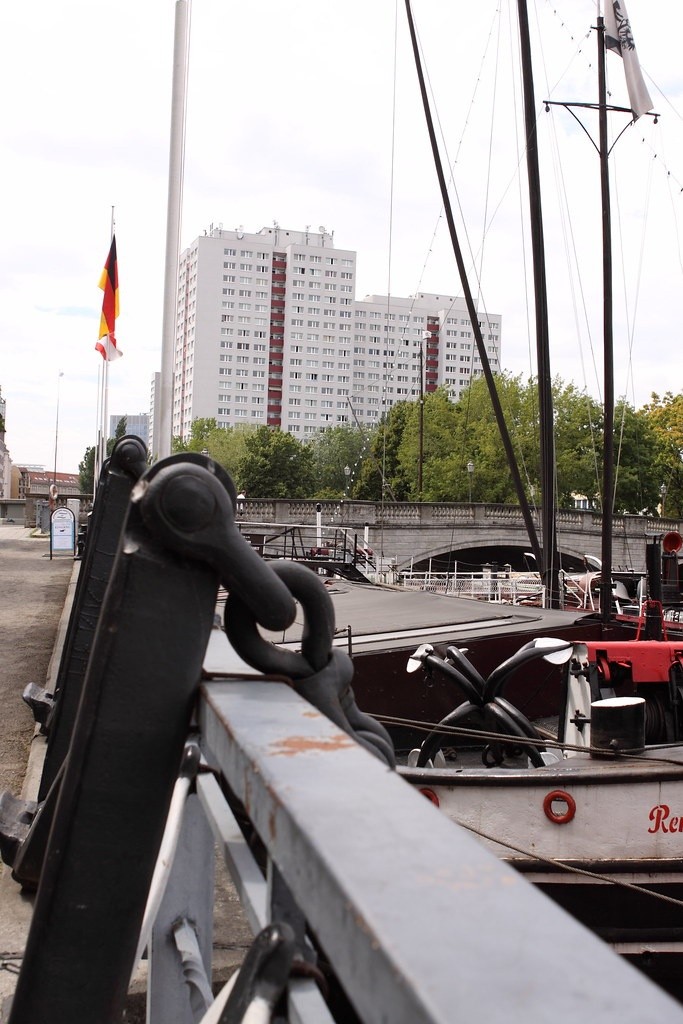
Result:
[94,233,123,363]
[602,0,654,125]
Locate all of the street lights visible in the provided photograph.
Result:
[344,464,351,499]
[467,461,474,502]
[661,484,666,518]
[54,372,64,485]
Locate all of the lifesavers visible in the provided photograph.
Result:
[49,484,59,500]
[420,789,439,807]
[543,790,577,824]
